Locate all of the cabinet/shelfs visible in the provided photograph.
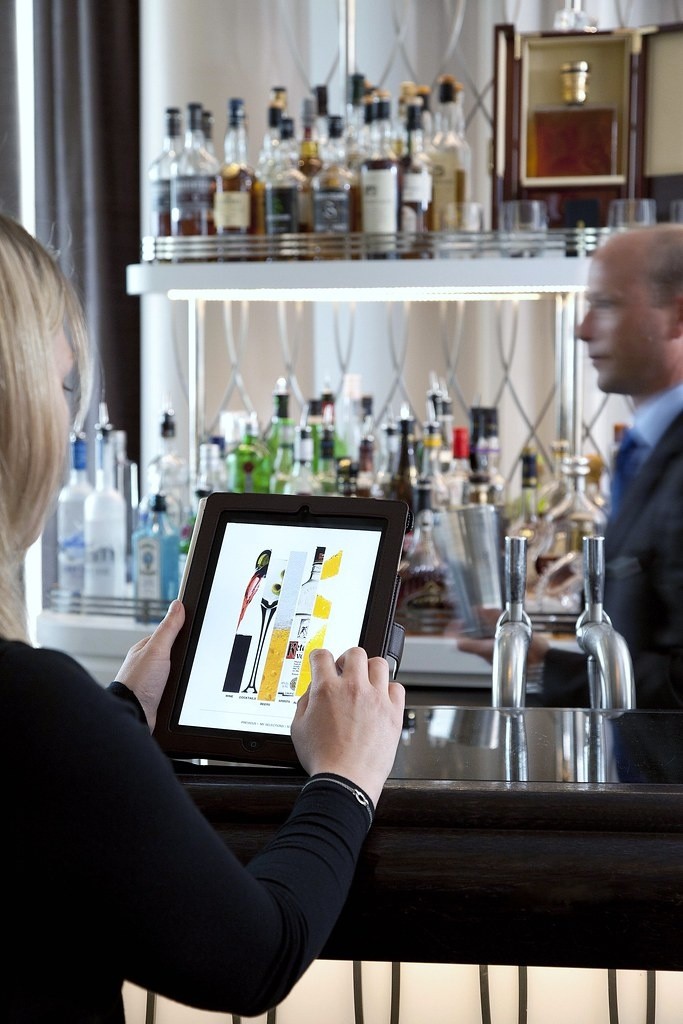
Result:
[35,228,604,709]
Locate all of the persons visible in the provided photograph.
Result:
[1,216,408,1024]
[455,223,683,712]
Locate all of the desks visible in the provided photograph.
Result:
[120,706,683,1024]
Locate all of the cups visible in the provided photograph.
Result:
[500,202,546,259]
[609,199,656,239]
[439,206,481,260]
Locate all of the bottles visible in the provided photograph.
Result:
[151,74,473,263]
[533,61,619,179]
[57,374,629,625]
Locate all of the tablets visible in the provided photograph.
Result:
[152,491,410,770]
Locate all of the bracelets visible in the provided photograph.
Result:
[299,779,373,835]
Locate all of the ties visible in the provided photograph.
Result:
[607,431,637,525]
